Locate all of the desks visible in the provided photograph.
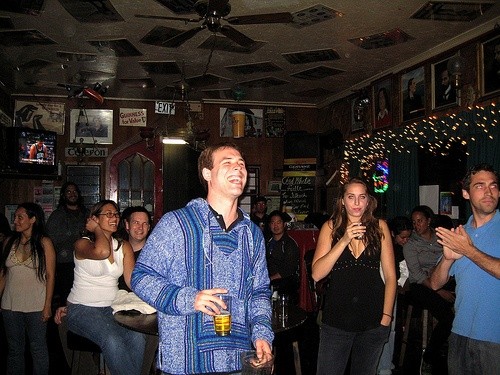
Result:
[115,307,308,375]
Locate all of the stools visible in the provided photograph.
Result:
[67,330,106,375]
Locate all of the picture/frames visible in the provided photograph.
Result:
[477,33,500,101]
[430,49,460,112]
[397,63,427,125]
[370,72,394,131]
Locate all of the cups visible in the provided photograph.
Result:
[231,111,246,138]
[353,223,365,240]
[212,296,232,336]
[278,294,289,319]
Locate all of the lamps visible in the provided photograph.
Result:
[64,83,107,105]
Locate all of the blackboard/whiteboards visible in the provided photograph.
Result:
[282,171,315,221]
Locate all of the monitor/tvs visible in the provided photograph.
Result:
[5,127,58,173]
[284,132,320,158]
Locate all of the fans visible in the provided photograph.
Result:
[135,0,294,47]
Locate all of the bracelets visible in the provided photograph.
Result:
[383,313,391,318]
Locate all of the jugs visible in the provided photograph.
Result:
[241,350,274,375]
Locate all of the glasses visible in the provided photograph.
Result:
[98,212,120,218]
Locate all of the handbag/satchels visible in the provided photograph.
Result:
[303,217,337,312]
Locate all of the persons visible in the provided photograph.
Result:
[45,182,91,307]
[250,196,271,240]
[0,203,57,375]
[379,206,456,375]
[54,199,160,375]
[19,136,51,160]
[432,162,500,375]
[265,209,300,308]
[130,142,274,375]
[376,87,391,127]
[402,77,423,113]
[311,179,397,375]
